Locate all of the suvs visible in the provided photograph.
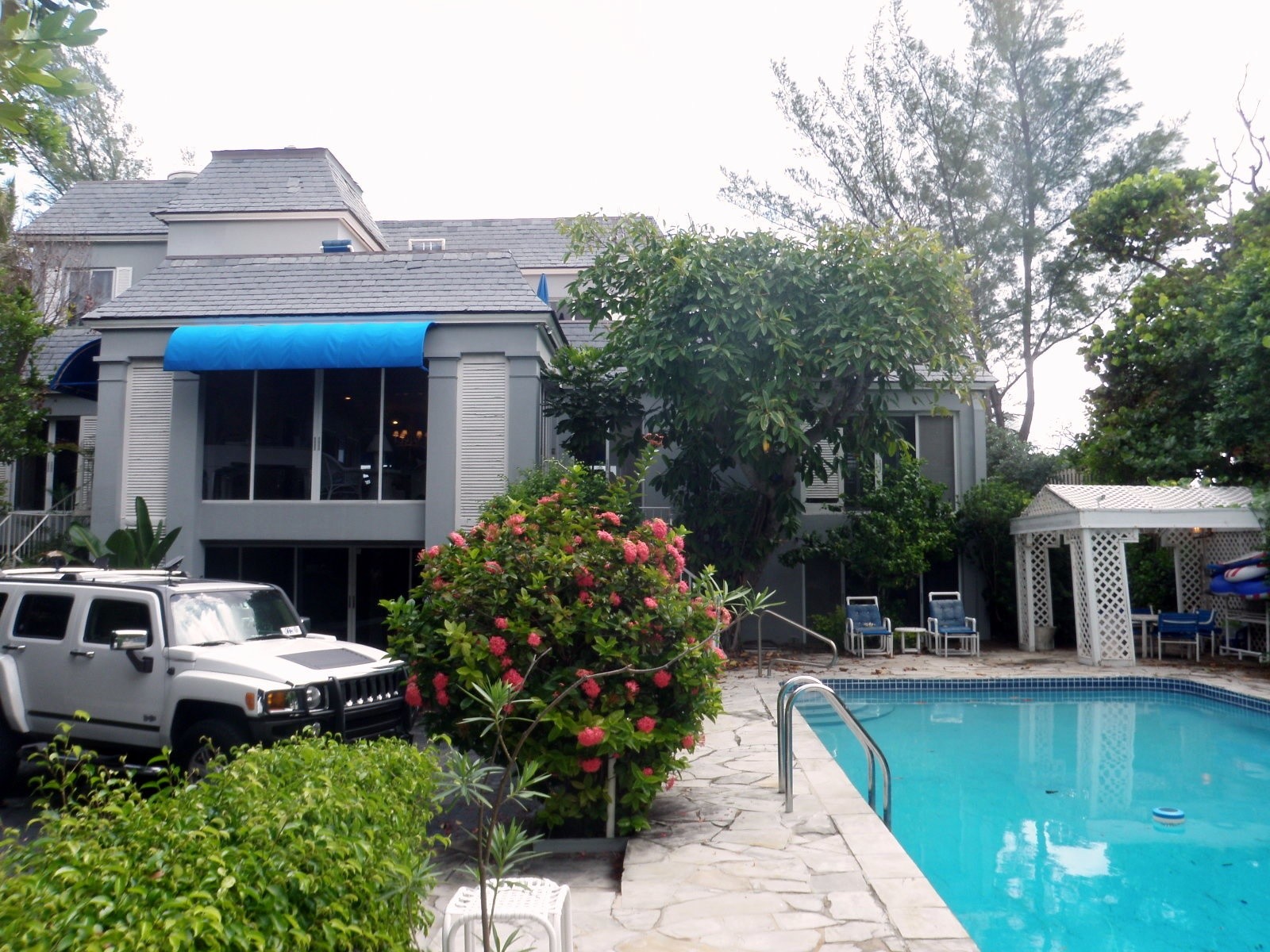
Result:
[0,555,416,798]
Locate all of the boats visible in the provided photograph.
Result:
[1206,551,1270,601]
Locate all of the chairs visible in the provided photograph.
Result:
[844,596,891,655]
[1131,604,1215,662]
[321,452,364,500]
[927,591,976,655]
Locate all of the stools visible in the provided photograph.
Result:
[939,630,980,657]
[857,631,893,659]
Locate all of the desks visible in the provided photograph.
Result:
[895,627,926,654]
[1131,614,1158,659]
[363,468,400,499]
